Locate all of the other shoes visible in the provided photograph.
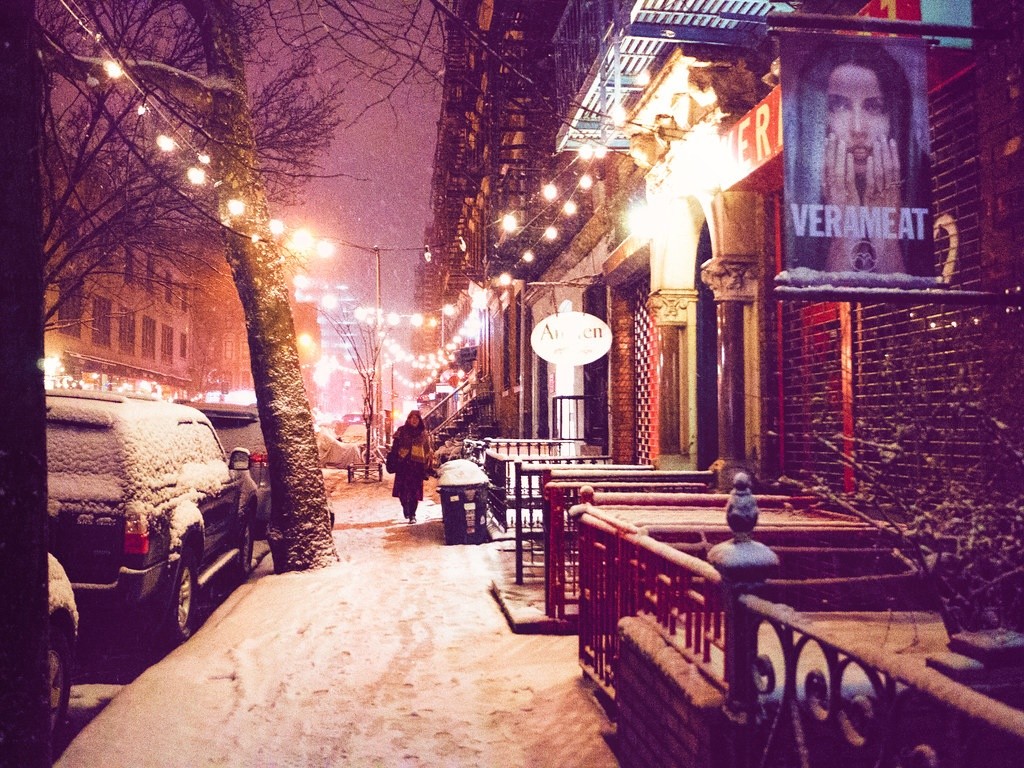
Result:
[409,515,416,524]
[403,507,410,518]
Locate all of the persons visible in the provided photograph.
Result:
[386,411,433,524]
[811,43,929,273]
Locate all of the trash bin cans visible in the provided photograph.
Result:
[435,458,489,544]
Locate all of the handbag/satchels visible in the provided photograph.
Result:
[387,450,399,473]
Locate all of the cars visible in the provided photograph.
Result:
[314,412,365,437]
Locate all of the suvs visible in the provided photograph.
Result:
[47,387,271,743]
